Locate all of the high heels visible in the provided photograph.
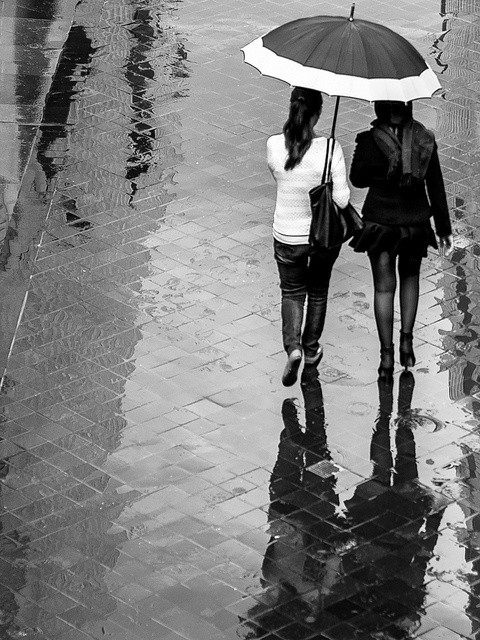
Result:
[378,342,394,379]
[399,330,415,371]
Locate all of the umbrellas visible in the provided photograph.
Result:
[236,3,445,139]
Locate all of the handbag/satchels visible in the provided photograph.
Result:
[308,182,364,249]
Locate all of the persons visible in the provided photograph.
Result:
[350,98,453,379]
[264,85,351,388]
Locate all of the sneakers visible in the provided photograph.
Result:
[282,349,302,386]
[305,344,323,365]
